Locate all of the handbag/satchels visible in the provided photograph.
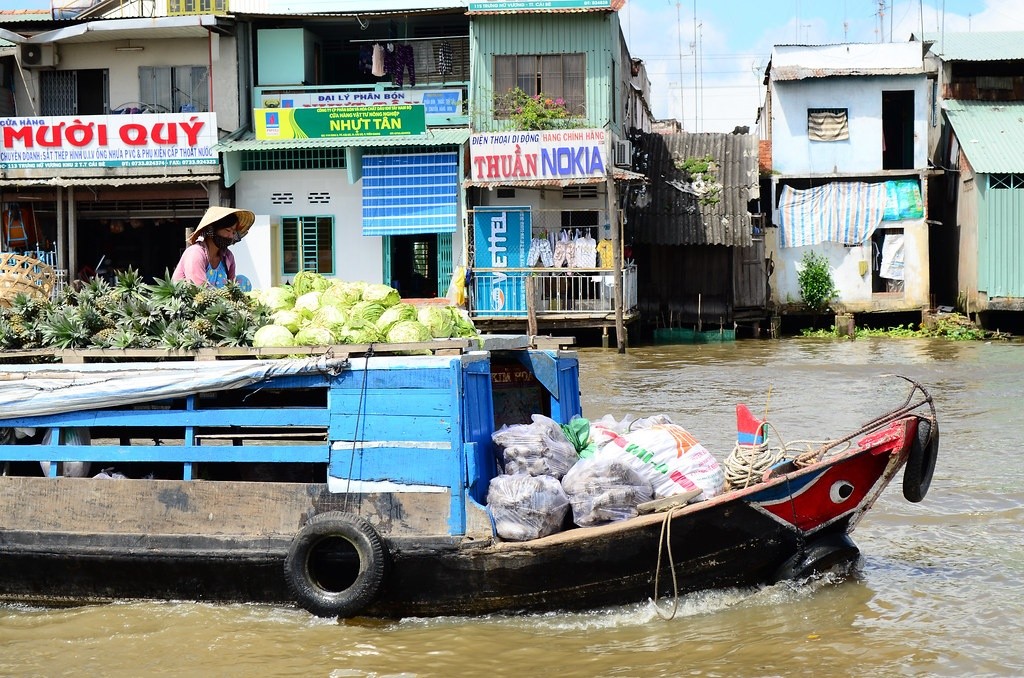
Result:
[488,413,674,540]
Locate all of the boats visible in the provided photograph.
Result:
[1,348,941,620]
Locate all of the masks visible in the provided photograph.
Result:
[213,233,233,249]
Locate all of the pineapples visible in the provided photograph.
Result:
[1,263,272,352]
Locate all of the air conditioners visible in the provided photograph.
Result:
[19,42,62,69]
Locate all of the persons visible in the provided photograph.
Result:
[170,205,255,291]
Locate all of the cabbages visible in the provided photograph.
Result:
[252,271,483,355]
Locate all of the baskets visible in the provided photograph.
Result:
[0,253,56,309]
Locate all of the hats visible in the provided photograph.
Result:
[186,206,255,245]
